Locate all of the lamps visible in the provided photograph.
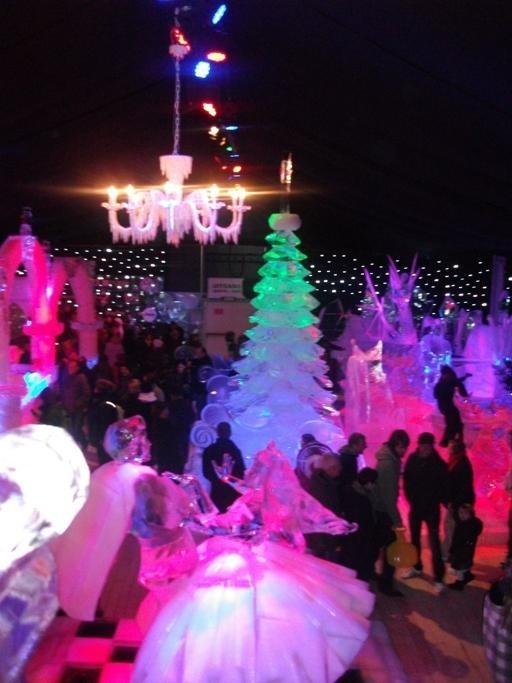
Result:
[98,19,254,246]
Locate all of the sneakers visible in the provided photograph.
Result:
[400,564,477,589]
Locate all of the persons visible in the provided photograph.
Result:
[201,417,247,514]
[432,363,473,447]
[474,573,512,683]
[291,426,479,596]
[14,314,249,475]
